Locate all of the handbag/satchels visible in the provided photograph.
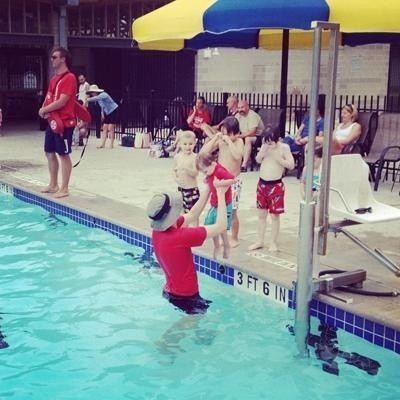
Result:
[121,132,153,149]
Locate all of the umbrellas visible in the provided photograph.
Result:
[134,0,400,139]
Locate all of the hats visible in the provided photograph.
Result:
[86,84,104,94]
[146,190,183,231]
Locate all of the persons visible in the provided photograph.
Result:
[165,94,362,260]
[146,174,230,354]
[39,47,77,198]
[76,74,119,149]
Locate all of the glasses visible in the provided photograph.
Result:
[51,55,60,59]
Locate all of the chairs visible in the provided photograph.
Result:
[136,98,400,192]
[314,151,400,224]
